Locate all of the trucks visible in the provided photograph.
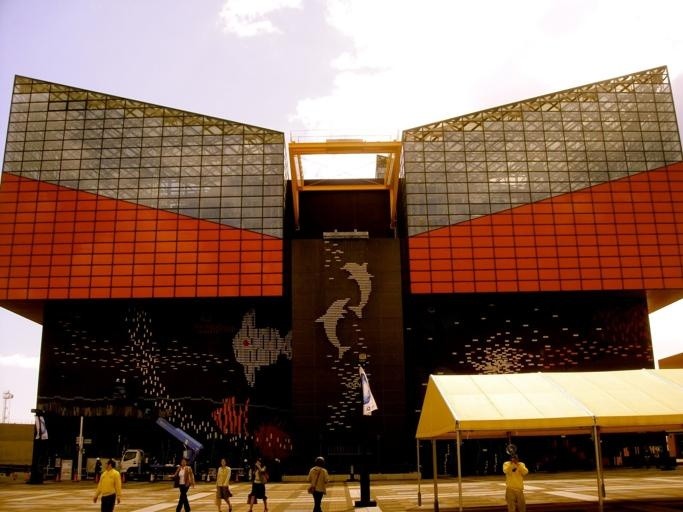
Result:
[119,449,245,484]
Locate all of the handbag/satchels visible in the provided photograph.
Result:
[307,486,315,494]
[262,473,269,483]
[246,493,257,504]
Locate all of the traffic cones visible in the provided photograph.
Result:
[24,472,30,481]
[206,474,211,482]
[56,474,61,482]
[120,473,128,483]
[234,472,241,482]
[73,472,79,482]
[12,472,17,480]
[94,474,100,483]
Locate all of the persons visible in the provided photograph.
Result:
[92,460,121,512]
[306,456,330,512]
[93,456,102,482]
[502,454,528,511]
[246,459,269,512]
[174,459,196,511]
[214,457,232,511]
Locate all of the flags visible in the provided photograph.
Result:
[358,366,378,415]
